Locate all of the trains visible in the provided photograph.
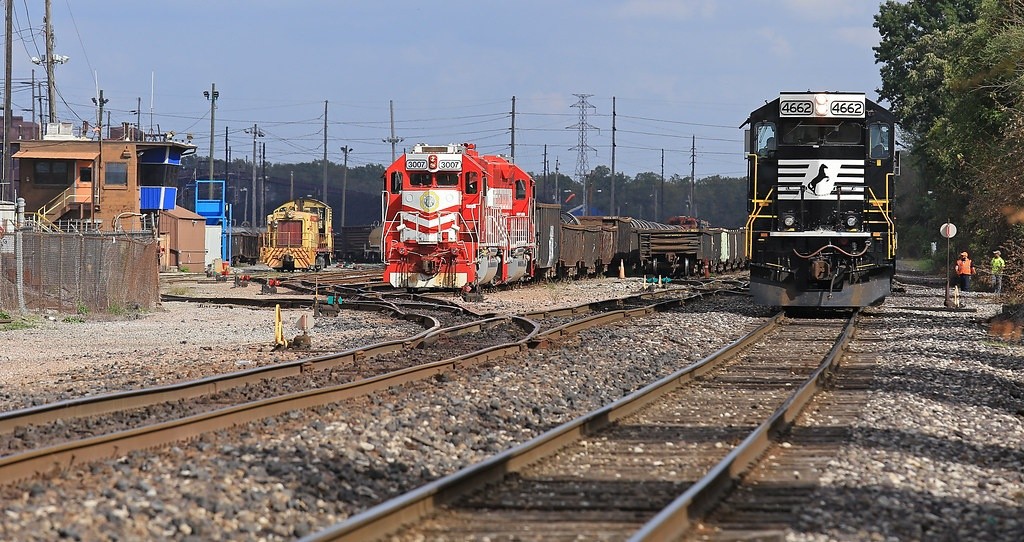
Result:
[379,143,707,292]
[738,89,903,308]
[259,186,384,272]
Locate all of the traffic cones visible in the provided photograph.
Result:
[619,258,626,279]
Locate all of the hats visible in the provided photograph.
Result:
[961,252,968,257]
[992,250,1001,256]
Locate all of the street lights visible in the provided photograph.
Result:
[31,53,69,123]
[380,137,405,164]
[244,124,265,228]
[91,90,110,140]
[339,145,354,234]
[203,83,219,200]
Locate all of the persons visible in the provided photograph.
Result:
[760,137,776,157]
[990,250,1006,292]
[955,252,976,291]
